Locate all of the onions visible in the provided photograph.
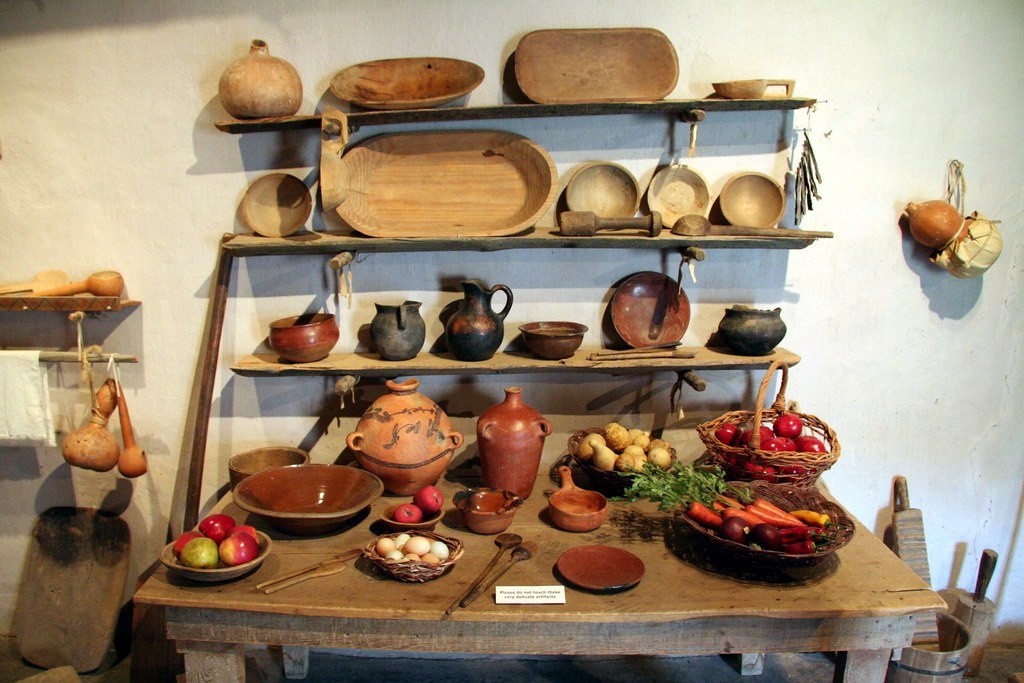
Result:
[716,516,782,551]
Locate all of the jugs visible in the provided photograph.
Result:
[445,279,514,361]
[370,299,425,361]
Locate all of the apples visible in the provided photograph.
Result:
[173,514,259,568]
[393,485,444,523]
[715,414,828,482]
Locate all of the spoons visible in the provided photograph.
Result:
[445,534,522,615]
[461,541,537,607]
[262,561,346,594]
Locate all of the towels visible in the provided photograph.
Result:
[0,349,57,448]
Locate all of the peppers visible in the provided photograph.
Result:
[776,510,847,554]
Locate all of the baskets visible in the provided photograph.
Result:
[680,481,856,558]
[364,530,465,583]
[696,361,841,488]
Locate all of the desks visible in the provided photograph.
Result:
[133,395,950,683]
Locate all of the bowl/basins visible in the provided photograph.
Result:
[269,313,339,363]
[720,171,785,229]
[518,321,588,360]
[233,463,384,536]
[646,166,709,229]
[565,160,641,228]
[718,305,787,356]
[452,488,524,535]
[228,446,311,490]
[712,79,767,99]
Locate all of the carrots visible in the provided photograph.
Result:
[684,495,805,526]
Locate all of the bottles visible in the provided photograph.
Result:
[346,378,465,496]
[476,383,551,499]
[218,38,304,118]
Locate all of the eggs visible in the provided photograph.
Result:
[376,533,449,563]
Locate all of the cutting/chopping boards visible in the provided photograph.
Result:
[11,505,130,673]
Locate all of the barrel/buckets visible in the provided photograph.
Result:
[885,611,972,682]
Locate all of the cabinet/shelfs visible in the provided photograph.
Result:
[213,96,832,408]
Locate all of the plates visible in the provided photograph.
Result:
[381,503,445,532]
[242,173,312,238]
[159,527,273,581]
[610,271,691,348]
[557,545,646,590]
[328,57,485,109]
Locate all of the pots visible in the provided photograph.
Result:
[549,465,609,532]
